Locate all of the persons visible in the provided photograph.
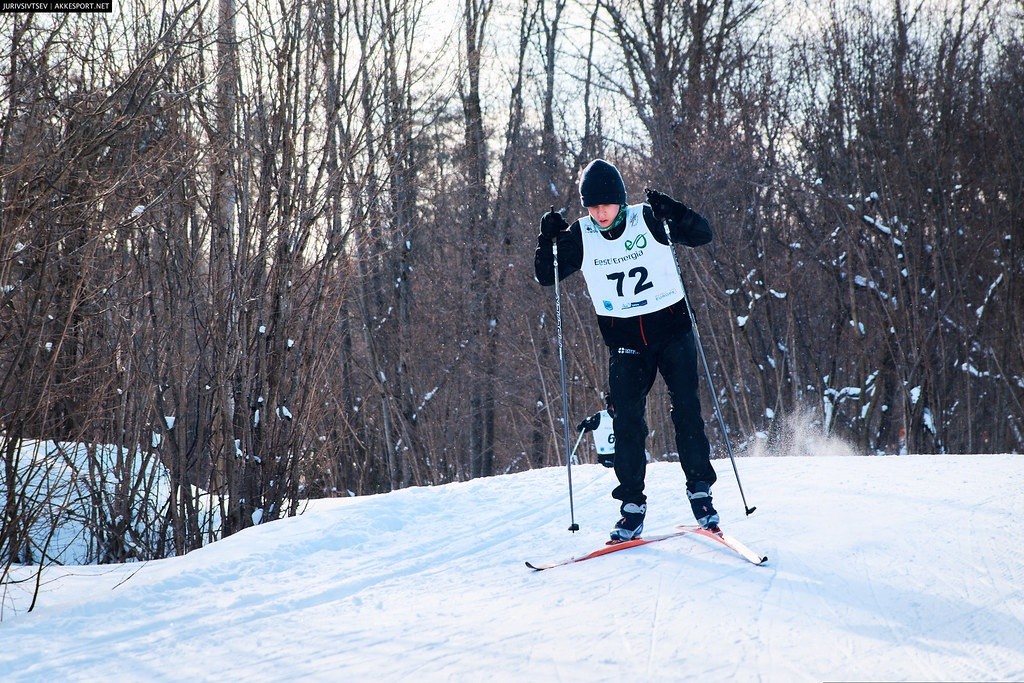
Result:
[574,390,650,469]
[533,158,720,542]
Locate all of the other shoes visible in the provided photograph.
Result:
[609,504,647,542]
[686,481,720,527]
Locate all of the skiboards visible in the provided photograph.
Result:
[524,523,773,570]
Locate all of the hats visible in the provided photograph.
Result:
[579,159,628,207]
[604,393,613,409]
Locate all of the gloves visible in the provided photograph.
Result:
[582,419,594,433]
[647,190,677,220]
[540,213,569,239]
